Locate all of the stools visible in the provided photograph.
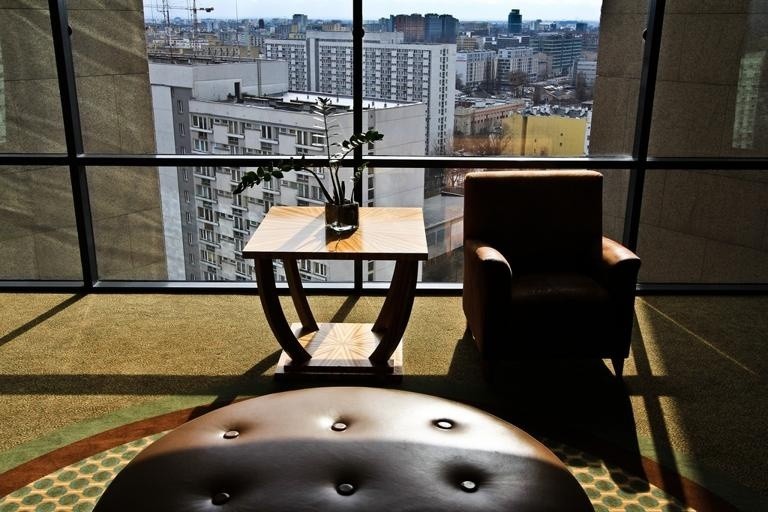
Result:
[92,386,593,512]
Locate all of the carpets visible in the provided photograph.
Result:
[0,379,768,512]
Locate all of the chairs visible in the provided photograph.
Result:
[463,168,642,386]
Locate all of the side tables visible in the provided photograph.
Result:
[242,206,428,380]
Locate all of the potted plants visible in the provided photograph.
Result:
[234,97,384,234]
